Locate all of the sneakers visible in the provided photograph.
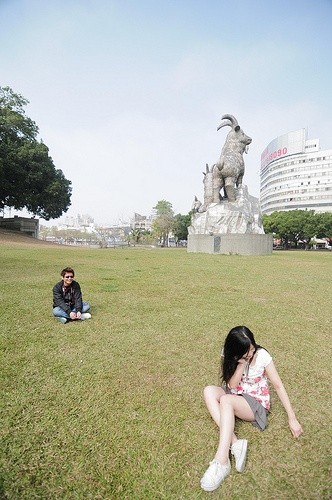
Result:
[232,438,248,473]
[200,459,232,492]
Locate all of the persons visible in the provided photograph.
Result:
[200,325,304,491]
[52,267,91,324]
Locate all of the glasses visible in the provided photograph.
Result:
[64,275,74,279]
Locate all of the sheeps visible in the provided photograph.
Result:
[212,114,252,203]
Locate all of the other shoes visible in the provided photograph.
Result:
[57,317,68,324]
[82,313,92,319]
